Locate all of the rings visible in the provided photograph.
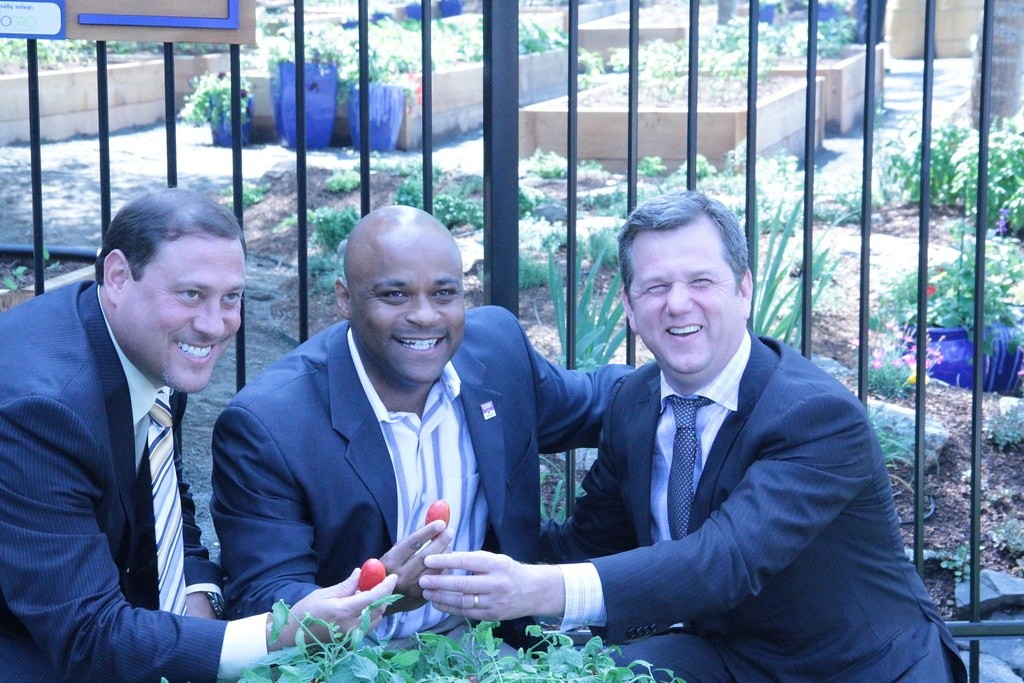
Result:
[474,595,478,607]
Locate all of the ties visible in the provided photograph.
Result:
[147,388,189,621]
[664,395,714,542]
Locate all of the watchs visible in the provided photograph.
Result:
[206,592,225,617]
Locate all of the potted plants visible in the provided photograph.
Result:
[257,18,352,151]
[333,38,419,153]
[869,171,1023,397]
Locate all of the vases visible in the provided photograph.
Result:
[207,93,254,148]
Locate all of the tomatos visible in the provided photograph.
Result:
[358,558,385,592]
[425,499,450,538]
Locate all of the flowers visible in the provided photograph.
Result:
[178,71,254,131]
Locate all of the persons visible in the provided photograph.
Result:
[208,207,636,670]
[0,188,398,683]
[419,193,967,683]
